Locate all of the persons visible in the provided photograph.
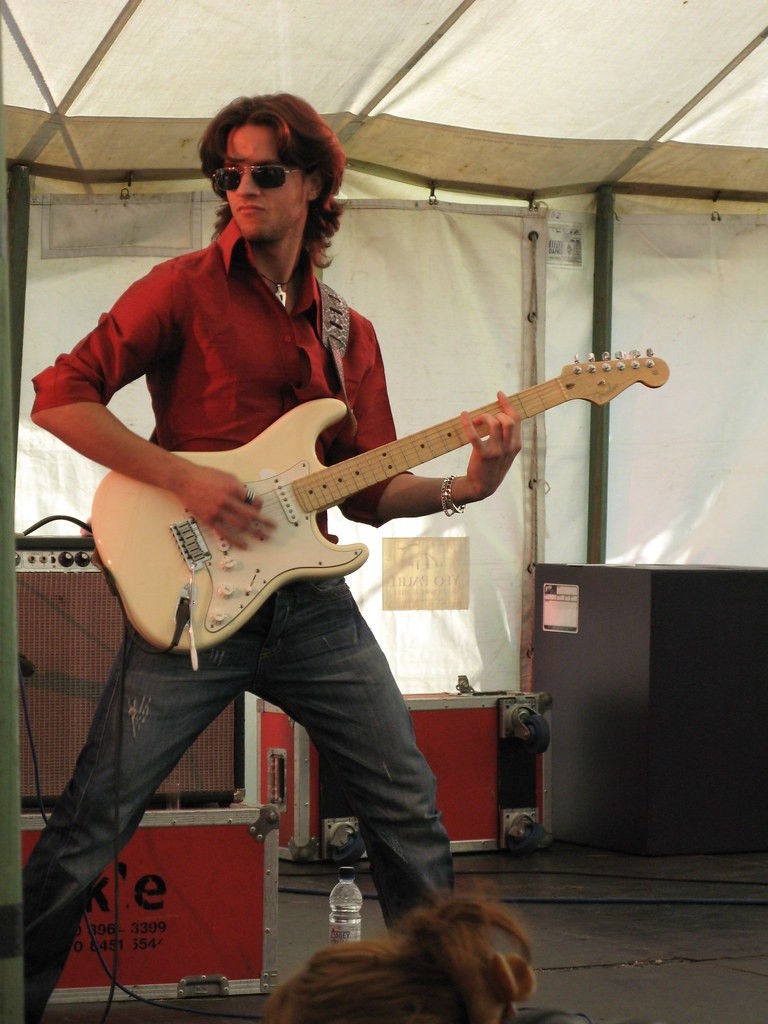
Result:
[261,884,537,1024]
[22,90,526,1023]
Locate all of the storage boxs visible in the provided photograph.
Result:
[20,804,280,1004]
[257,694,556,865]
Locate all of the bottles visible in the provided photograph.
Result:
[327,865,363,946]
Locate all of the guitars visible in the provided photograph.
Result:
[91,349,670,656]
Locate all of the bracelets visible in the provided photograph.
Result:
[440,475,466,517]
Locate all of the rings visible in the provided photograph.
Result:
[244,488,256,505]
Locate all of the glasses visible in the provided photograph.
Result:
[212,166,305,191]
